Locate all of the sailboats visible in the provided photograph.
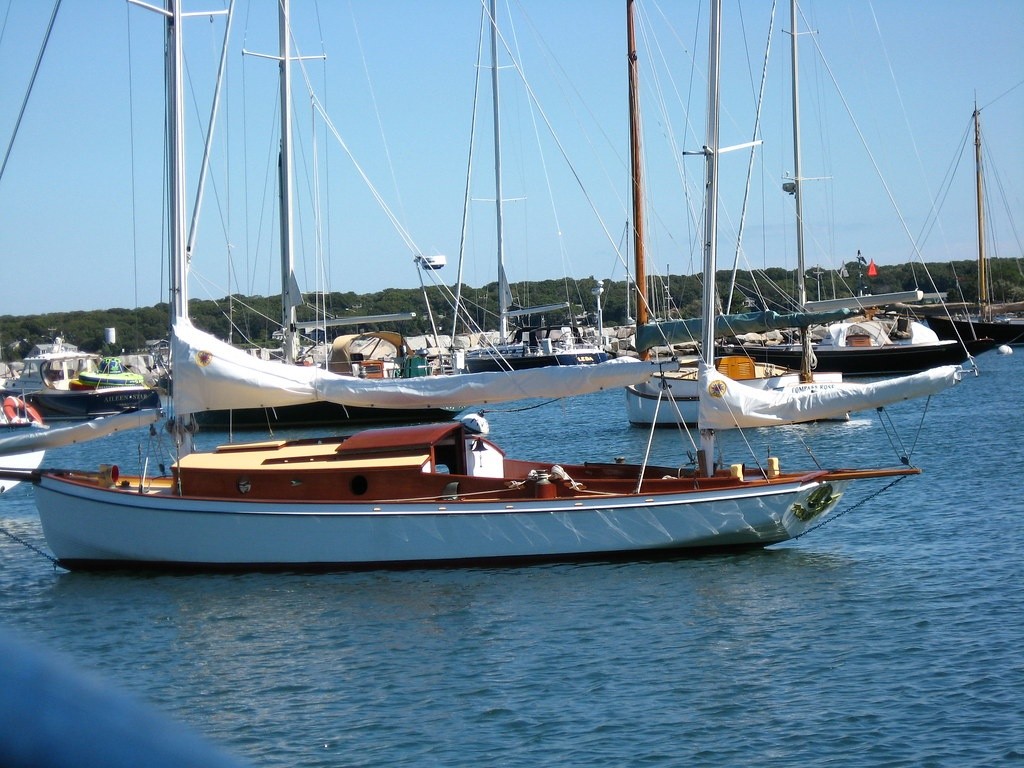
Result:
[0,2,1024,568]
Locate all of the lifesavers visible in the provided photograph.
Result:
[3,395,43,425]
